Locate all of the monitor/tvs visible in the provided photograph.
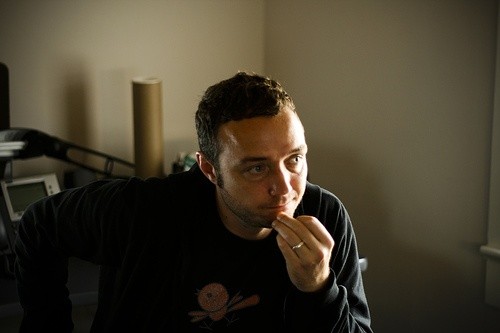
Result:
[1,173,61,223]
